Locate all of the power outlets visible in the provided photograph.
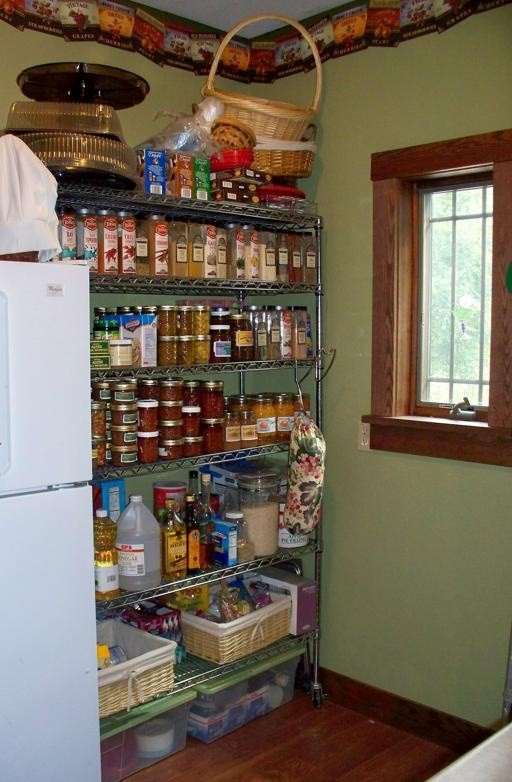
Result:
[358,420,369,452]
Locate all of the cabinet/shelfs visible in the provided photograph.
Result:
[40,182,322,781]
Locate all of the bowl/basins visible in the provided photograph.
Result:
[210,150,255,172]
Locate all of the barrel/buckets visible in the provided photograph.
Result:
[113,495,161,592]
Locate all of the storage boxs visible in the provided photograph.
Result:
[175,634,308,745]
[242,567,318,636]
[100,688,197,780]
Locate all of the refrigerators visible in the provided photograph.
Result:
[0,261,102,782]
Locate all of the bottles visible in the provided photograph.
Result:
[244,305,314,361]
[89,377,312,471]
[93,466,312,601]
[56,205,317,284]
[92,302,254,368]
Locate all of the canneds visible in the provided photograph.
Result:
[93,305,256,370]
[91,377,310,471]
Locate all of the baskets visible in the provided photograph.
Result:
[96,619,178,720]
[176,582,292,665]
[201,12,323,179]
